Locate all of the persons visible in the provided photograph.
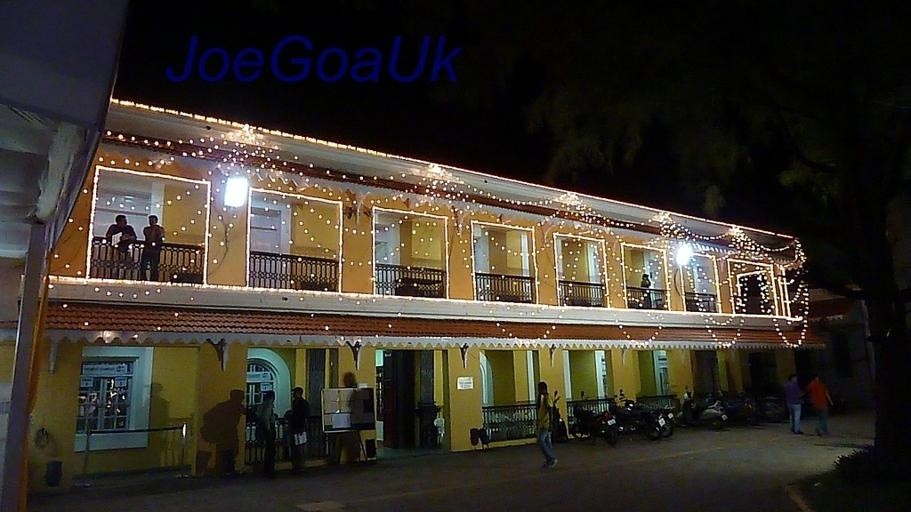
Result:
[785,373,806,435]
[253,390,280,478]
[806,374,834,439]
[139,215,165,282]
[105,216,136,280]
[285,387,311,476]
[534,381,557,470]
[639,273,652,308]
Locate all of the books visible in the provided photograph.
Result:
[109,231,122,246]
[293,431,307,446]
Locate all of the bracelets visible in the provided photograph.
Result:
[119,236,122,241]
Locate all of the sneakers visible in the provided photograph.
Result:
[542,456,560,470]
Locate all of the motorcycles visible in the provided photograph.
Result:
[569,385,788,444]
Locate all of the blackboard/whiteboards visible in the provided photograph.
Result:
[321,386,377,433]
